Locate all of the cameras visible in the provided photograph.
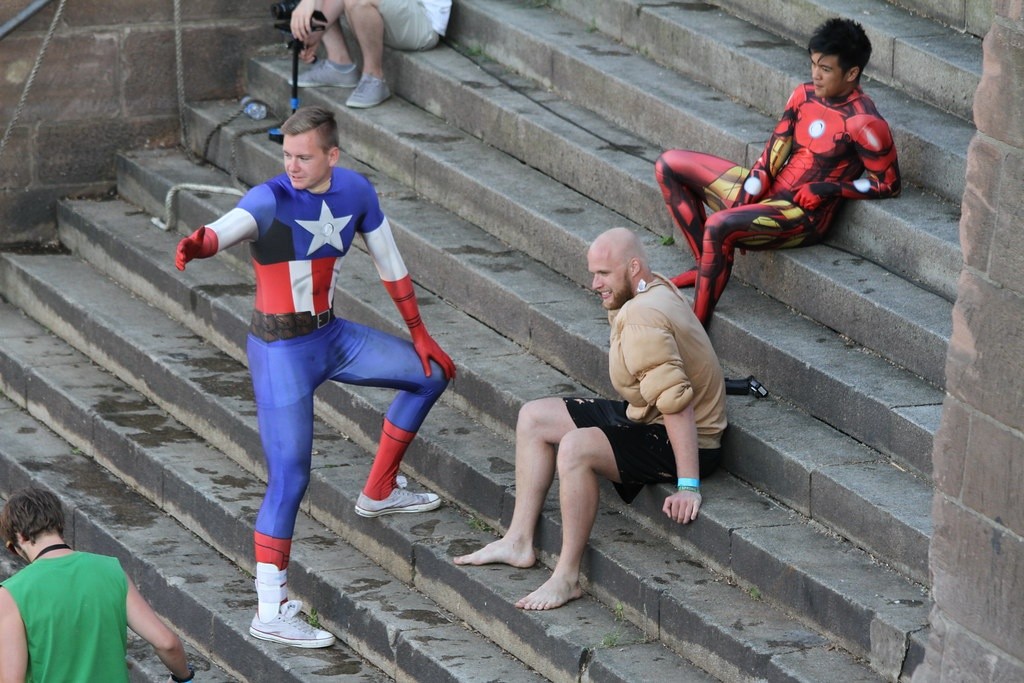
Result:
[271,0,300,20]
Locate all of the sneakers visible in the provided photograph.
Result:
[355,474,441,517]
[346,72,390,108]
[248,598,335,649]
[287,58,359,89]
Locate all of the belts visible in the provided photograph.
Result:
[311,310,333,329]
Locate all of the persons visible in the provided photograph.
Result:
[0,488,192,683]
[174,109,458,648]
[288,0,453,109]
[655,17,902,331]
[452,227,729,610]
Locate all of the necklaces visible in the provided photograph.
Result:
[32,544,71,563]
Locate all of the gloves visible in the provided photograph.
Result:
[790,181,841,210]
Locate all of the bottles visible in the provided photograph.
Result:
[241,96,267,119]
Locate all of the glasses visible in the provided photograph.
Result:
[6,541,18,555]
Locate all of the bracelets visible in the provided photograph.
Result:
[677,478,700,493]
[170,665,195,683]
